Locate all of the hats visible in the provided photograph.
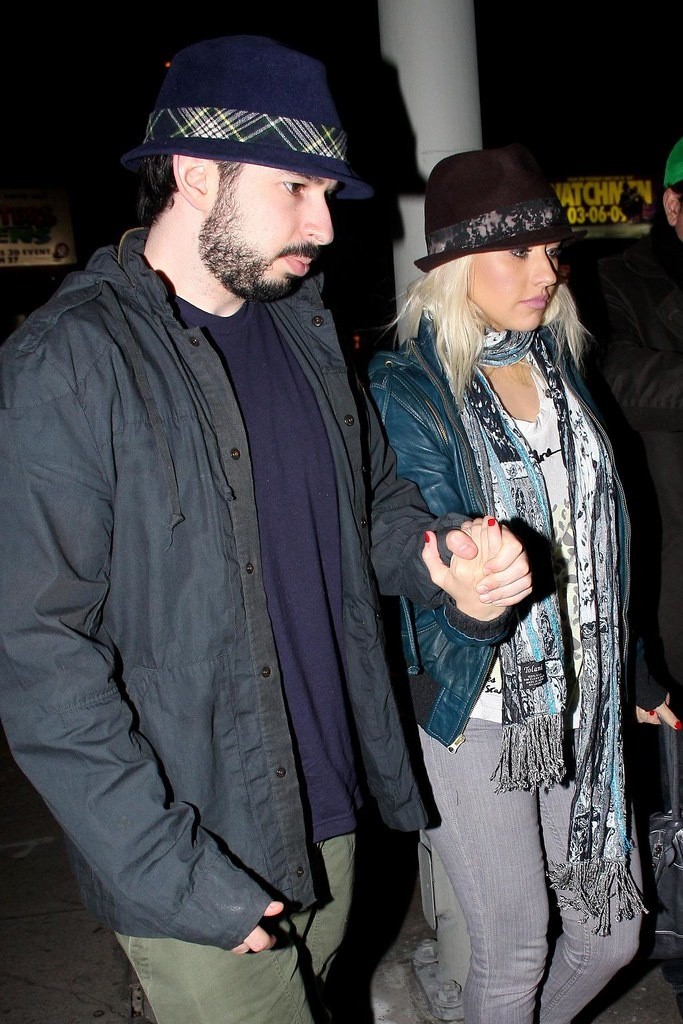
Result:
[404,143,589,273]
[663,137,683,189]
[121,34,375,200]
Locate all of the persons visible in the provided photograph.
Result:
[0,34,533,1024]
[590,138,683,722]
[618,183,655,225]
[365,145,683,1024]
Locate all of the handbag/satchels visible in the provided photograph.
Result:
[647,712,683,960]
